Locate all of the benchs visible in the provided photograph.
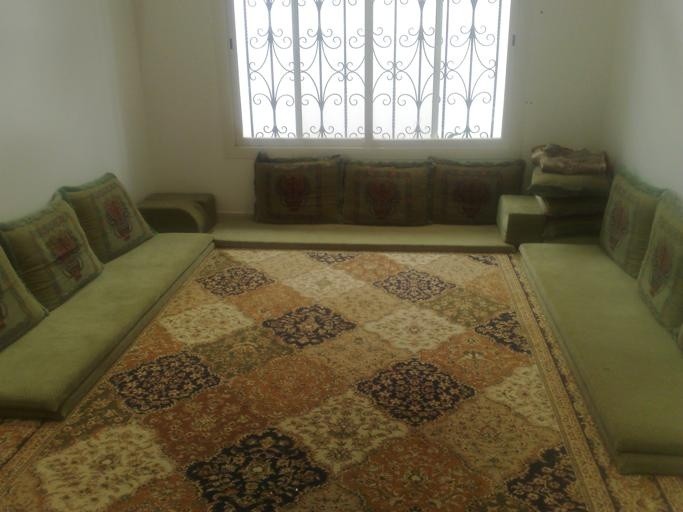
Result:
[0,232,217,420]
[518,241,683,475]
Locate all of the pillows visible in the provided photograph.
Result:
[635,190,683,341]
[336,157,435,225]
[528,143,611,221]
[252,149,344,225]
[0,172,154,351]
[599,172,669,278]
[429,153,527,225]
